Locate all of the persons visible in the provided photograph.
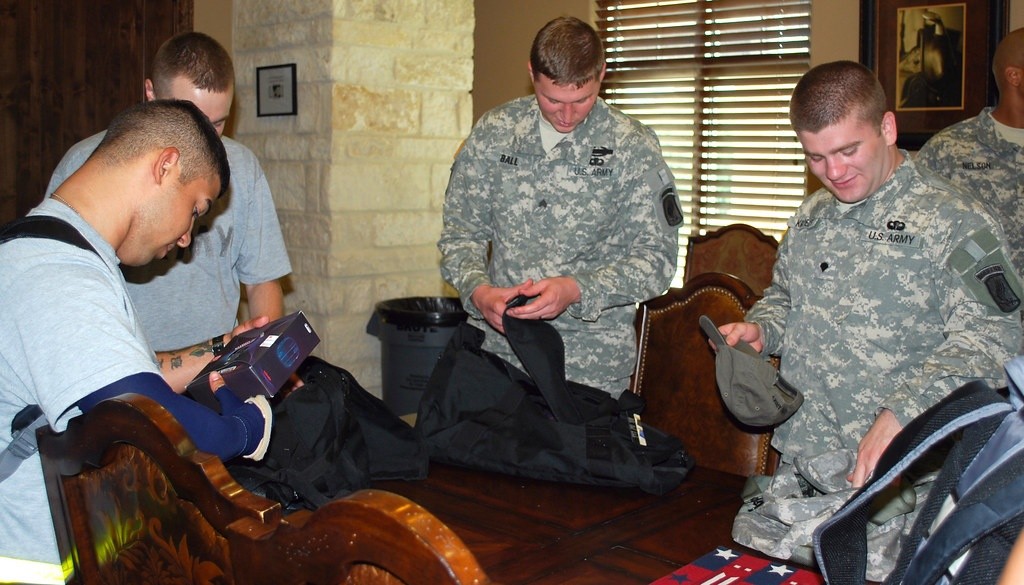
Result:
[437,18,684,399]
[709,61,1024,490]
[44,32,305,397]
[913,26,1024,279]
[1,99,305,585]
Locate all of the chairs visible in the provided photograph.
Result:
[628,223,783,479]
[33,390,492,585]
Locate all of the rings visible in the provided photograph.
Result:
[539,316,541,319]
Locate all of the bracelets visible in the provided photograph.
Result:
[212,334,225,357]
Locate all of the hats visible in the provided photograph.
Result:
[700,315,803,427]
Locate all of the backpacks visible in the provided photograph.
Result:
[812,357,1024,585]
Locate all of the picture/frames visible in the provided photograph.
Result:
[254,63,299,119]
[860,0,1011,152]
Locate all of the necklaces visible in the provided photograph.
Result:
[51,193,81,217]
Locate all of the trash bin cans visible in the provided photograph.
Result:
[365,296,469,417]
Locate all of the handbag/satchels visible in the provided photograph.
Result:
[222,359,428,508]
[414,294,697,496]
[729,453,940,582]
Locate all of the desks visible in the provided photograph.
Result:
[364,458,828,585]
